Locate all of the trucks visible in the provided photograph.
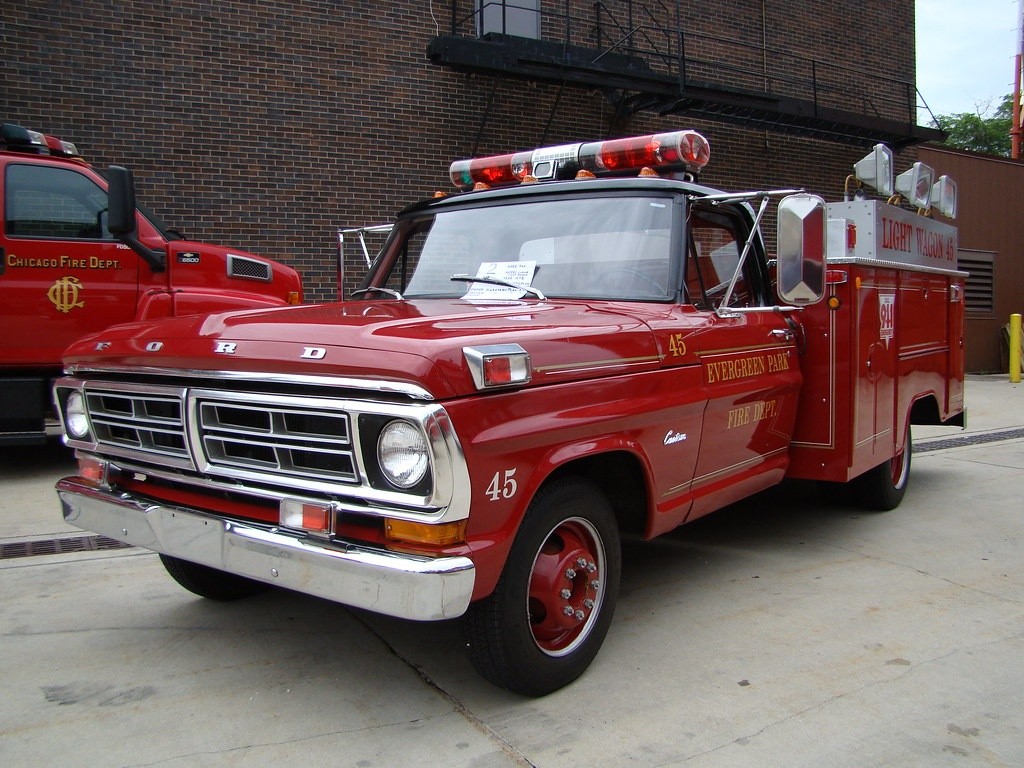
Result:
[0,122,303,467]
[51,128,970,699]
